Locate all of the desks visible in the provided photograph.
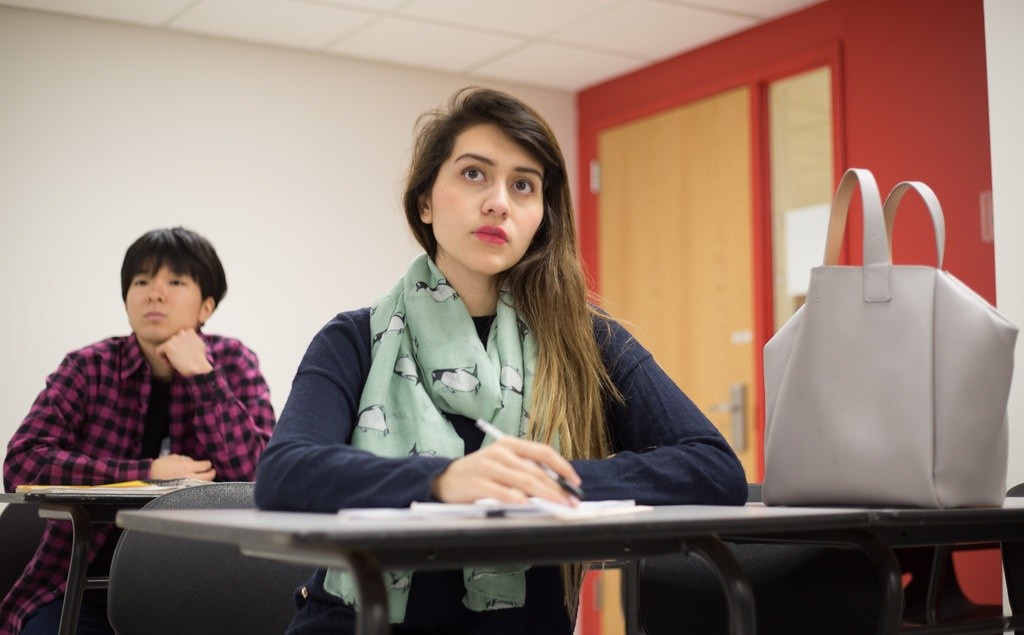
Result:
[0,492,157,635]
[116,494,1024,635]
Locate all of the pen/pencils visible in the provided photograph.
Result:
[476,418,587,500]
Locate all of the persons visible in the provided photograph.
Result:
[1,226,278,635]
[254,89,749,635]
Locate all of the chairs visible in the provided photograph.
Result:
[623,482,906,635]
[102,481,321,635]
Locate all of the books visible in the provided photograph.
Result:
[14,479,219,495]
[340,497,655,518]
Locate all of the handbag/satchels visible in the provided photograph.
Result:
[762,168,1017,509]
